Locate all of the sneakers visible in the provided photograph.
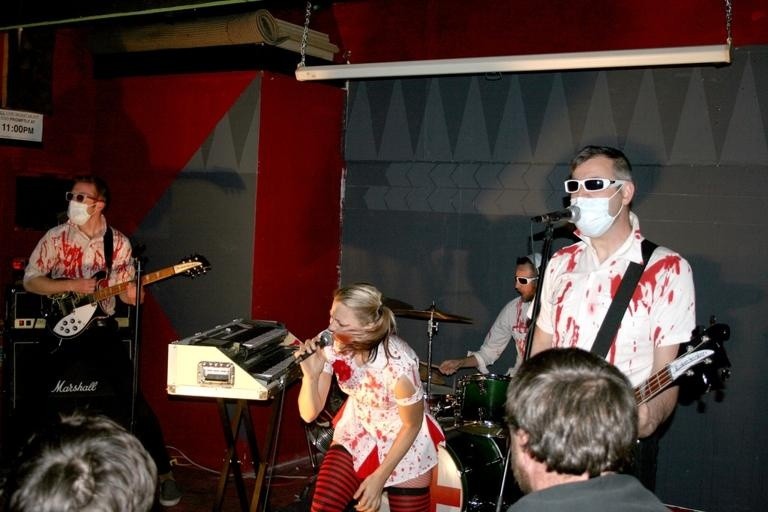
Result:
[157,473,185,506]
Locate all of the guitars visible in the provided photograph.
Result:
[47,251,213,341]
[634,315,731,414]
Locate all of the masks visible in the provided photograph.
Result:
[66,201,99,225]
[569,187,626,238]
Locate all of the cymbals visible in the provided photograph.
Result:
[393,307,474,324]
[419,363,447,386]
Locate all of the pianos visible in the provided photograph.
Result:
[165,316,308,402]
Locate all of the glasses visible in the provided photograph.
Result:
[561,177,626,194]
[514,276,536,286]
[65,191,98,203]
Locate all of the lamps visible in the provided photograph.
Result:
[294,41,734,81]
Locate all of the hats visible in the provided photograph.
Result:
[522,252,544,275]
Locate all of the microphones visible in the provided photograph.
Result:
[131,243,146,258]
[287,331,334,370]
[528,205,582,224]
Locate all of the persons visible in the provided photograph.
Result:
[0,414,159,512]
[299,285,444,511]
[21,176,184,508]
[439,250,542,378]
[526,144,696,496]
[503,347,670,512]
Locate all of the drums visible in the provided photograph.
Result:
[429,429,506,512]
[427,393,457,421]
[453,373,514,437]
